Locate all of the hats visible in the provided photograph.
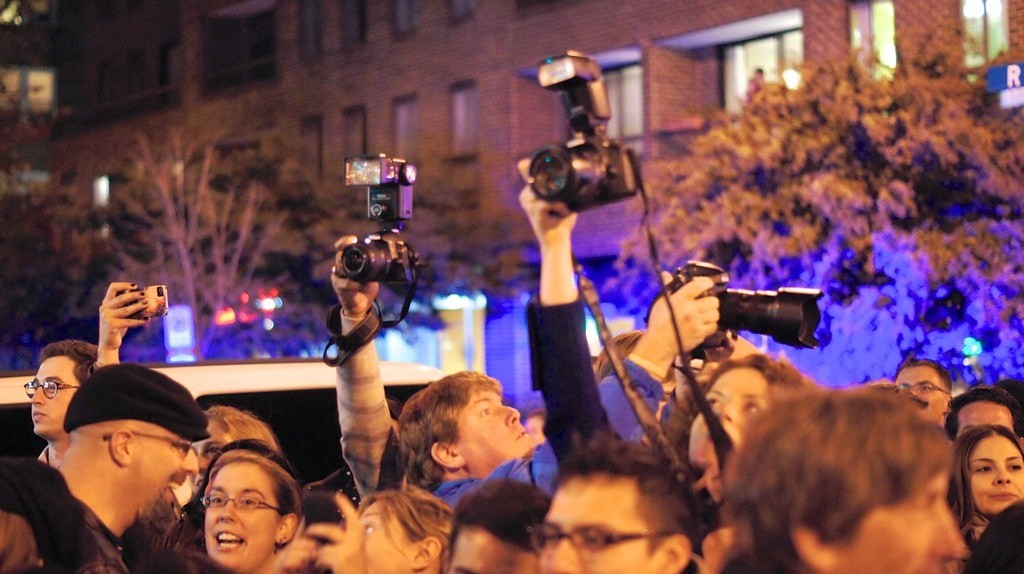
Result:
[63,362,211,442]
[0,455,99,574]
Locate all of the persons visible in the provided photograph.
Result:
[747,68,767,105]
[0,158,1023,574]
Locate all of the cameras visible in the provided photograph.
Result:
[526,49,640,215]
[335,153,428,282]
[645,261,825,372]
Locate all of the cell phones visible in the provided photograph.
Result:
[302,492,338,544]
[116,285,168,319]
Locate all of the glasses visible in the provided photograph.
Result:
[104,430,203,460]
[200,495,288,516]
[525,521,671,557]
[897,380,944,395]
[22,380,80,400]
